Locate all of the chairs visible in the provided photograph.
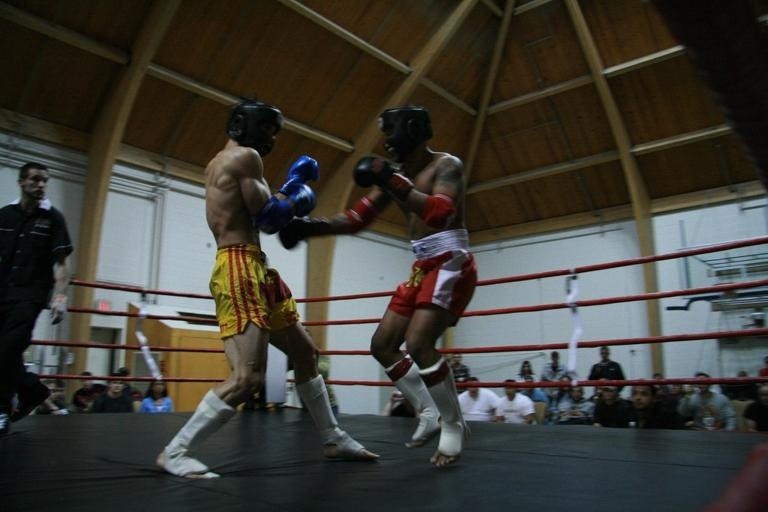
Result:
[132,400,143,412]
[534,401,548,424]
[730,398,755,432]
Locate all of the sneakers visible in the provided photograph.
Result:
[0,381,51,436]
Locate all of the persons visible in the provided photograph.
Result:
[382,345,768,433]
[0,161,74,440]
[155,98,381,481]
[277,104,477,471]
[26,366,174,416]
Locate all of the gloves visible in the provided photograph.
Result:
[353,155,414,202]
[290,181,317,216]
[286,155,320,184]
[278,216,329,250]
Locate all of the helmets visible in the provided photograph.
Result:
[378,105,434,157]
[226,101,283,156]
